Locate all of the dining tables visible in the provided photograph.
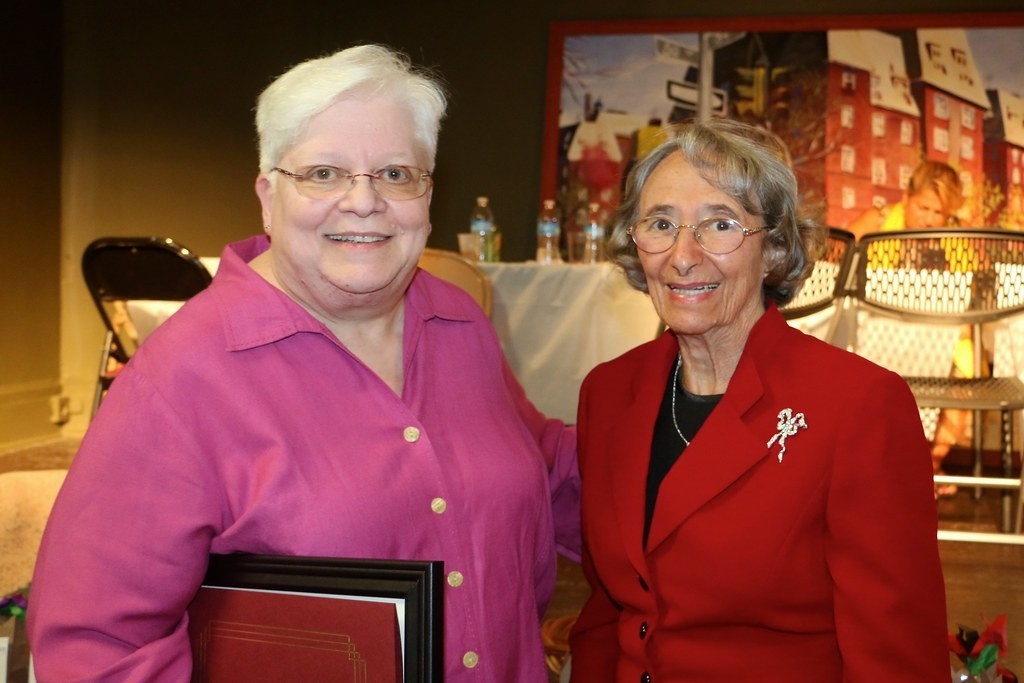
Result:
[123,251,669,427]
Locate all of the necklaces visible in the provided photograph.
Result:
[672,353,691,445]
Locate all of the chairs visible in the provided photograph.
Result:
[81,234,216,424]
[816,248,1023,536]
[417,247,493,323]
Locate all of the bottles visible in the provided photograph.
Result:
[471,198,501,264]
[536,199,563,264]
[581,203,604,264]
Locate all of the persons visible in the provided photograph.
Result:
[571,118,953,681]
[22,43,575,681]
[837,160,992,271]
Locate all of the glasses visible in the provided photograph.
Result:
[624,216,774,255]
[272,165,435,202]
